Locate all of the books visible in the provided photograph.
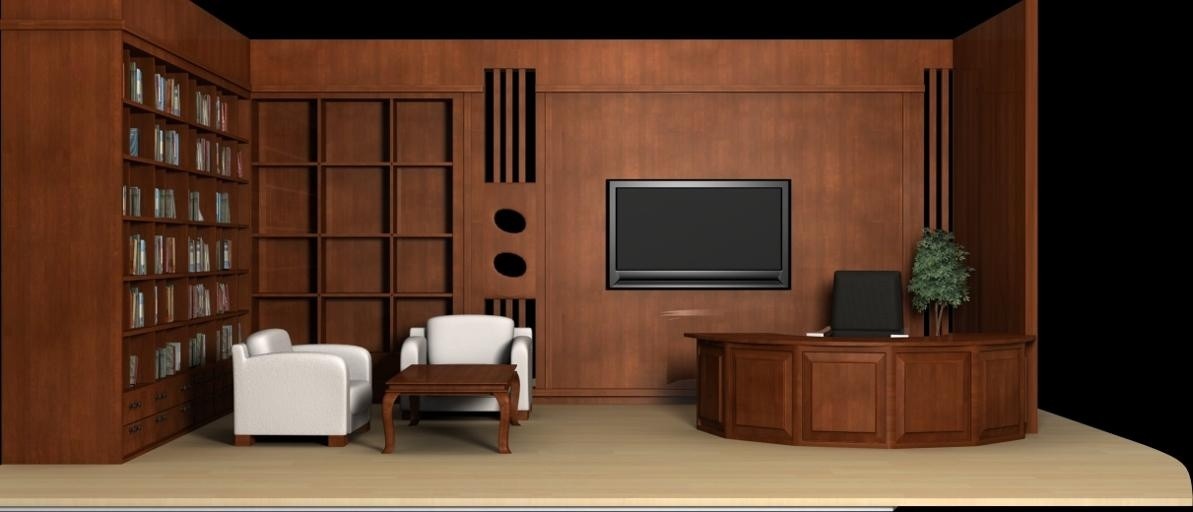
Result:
[122,58,247,392]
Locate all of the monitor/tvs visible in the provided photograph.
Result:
[610,181,789,289]
